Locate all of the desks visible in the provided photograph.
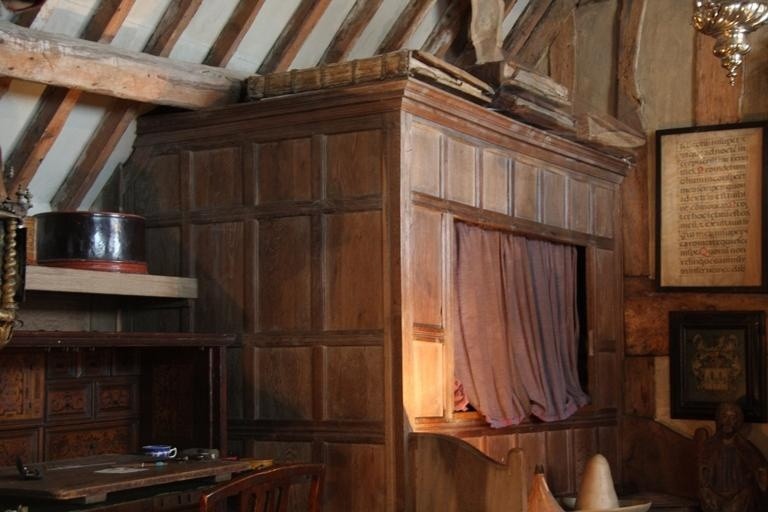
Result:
[0,451,273,512]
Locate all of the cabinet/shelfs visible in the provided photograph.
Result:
[0,263,238,458]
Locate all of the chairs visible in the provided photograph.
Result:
[201,463,325,512]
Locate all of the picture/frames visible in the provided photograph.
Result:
[668,309,768,425]
[654,120,768,294]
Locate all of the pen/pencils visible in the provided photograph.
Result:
[111,461,169,468]
[219,456,240,462]
[170,455,205,462]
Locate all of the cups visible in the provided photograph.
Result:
[142,443,178,461]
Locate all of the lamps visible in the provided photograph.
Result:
[690,0,767,88]
